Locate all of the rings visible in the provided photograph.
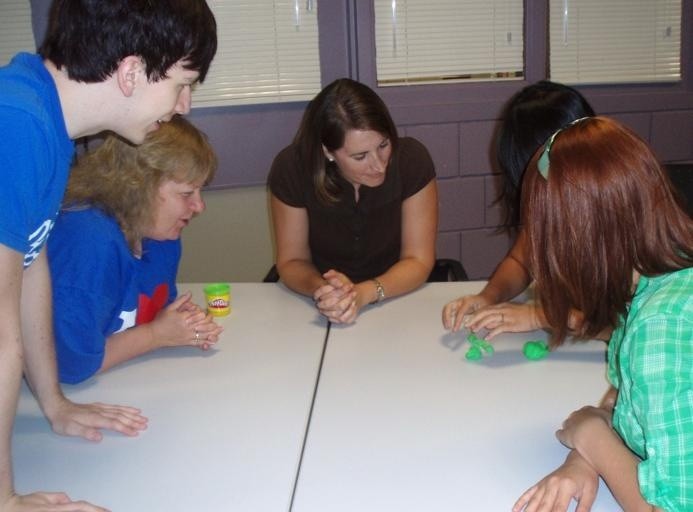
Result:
[500,312,503,322]
[195,331,198,340]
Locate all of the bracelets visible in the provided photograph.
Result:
[369,279,384,304]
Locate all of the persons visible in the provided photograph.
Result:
[267,78,438,323]
[0,1,218,512]
[46,113,223,385]
[508,116,693,511]
[441,80,615,341]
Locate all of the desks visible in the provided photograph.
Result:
[11,279,623,511]
